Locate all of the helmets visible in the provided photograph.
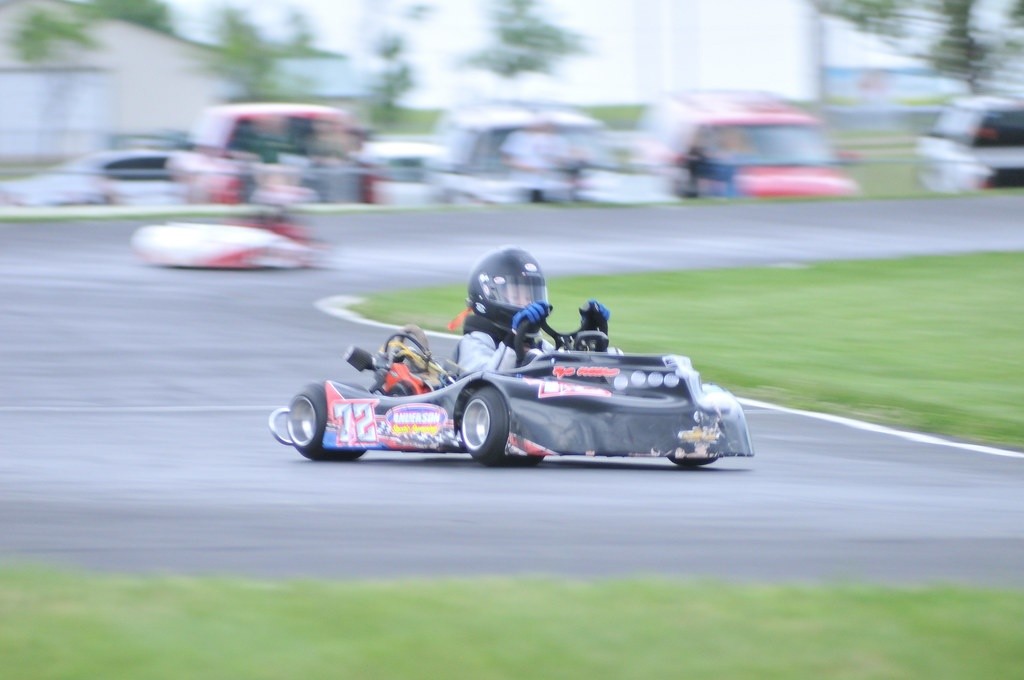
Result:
[465,249,546,334]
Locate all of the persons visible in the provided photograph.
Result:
[170,128,374,204]
[440,250,610,389]
[501,107,587,204]
[670,147,734,200]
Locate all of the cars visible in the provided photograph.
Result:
[425,103,621,204]
[0,149,171,206]
[659,107,858,196]
[916,96,1024,193]
[168,103,380,205]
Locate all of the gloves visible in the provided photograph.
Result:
[581,299,610,328]
[512,300,550,333]
